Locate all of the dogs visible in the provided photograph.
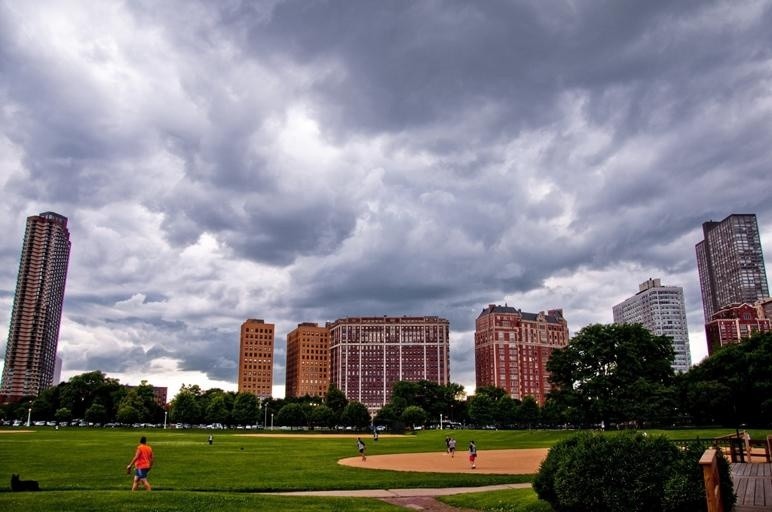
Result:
[10,474,40,491]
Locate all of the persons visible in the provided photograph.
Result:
[741,431,752,451]
[469,440,477,468]
[373,428,378,441]
[449,437,456,458]
[208,433,214,445]
[358,440,367,461]
[127,436,155,491]
[358,437,360,447]
[445,435,451,453]
